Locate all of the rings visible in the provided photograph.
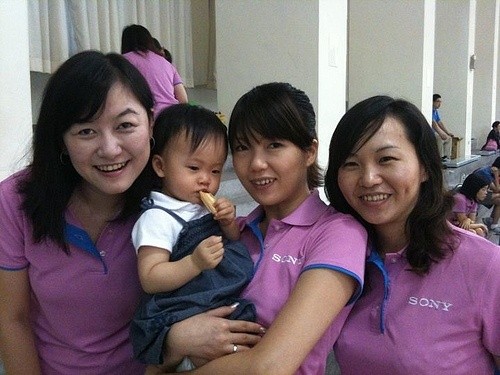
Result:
[233,344,237,353]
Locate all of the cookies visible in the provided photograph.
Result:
[199,191,216,214]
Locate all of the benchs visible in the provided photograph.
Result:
[442,155,481,191]
[476,149,500,166]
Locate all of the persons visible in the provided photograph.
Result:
[324,96,499,375]
[0,50,154,375]
[142,83,370,375]
[431,93,455,161]
[472,156,500,235]
[446,173,490,238]
[486,121,500,152]
[131,102,259,369]
[121,24,188,124]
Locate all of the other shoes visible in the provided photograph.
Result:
[482,217,493,230]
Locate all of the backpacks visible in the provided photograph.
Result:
[483,138,497,151]
